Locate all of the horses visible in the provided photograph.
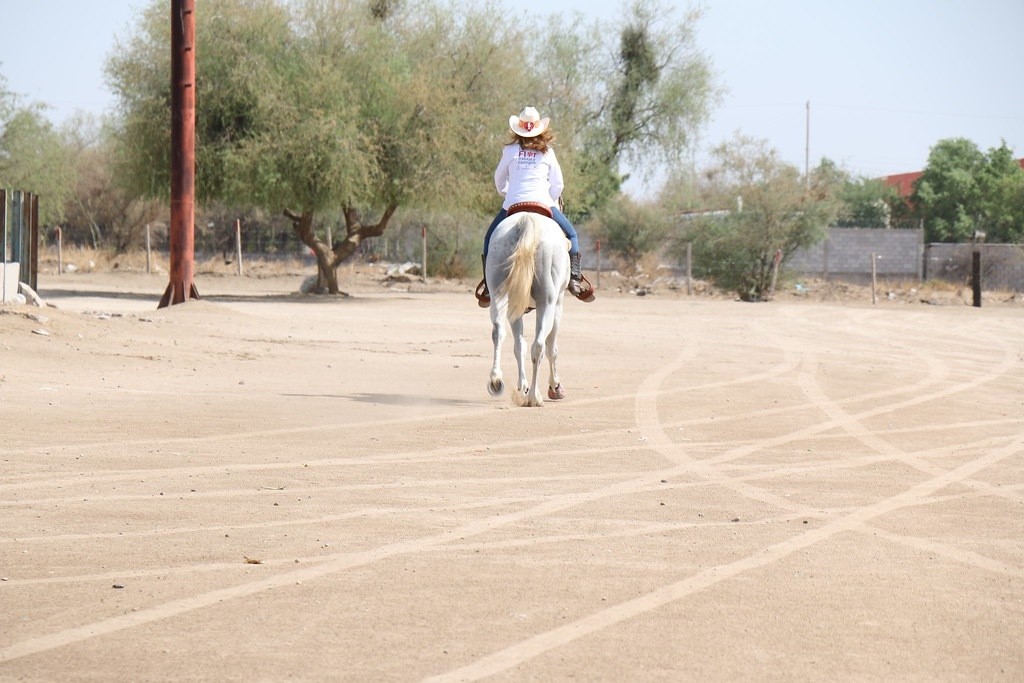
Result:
[484,194,571,408]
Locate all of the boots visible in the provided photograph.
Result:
[481,254,490,297]
[571,252,584,295]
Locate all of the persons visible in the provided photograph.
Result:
[477,107,596,308]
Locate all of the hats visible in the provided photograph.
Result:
[509,106,550,137]
